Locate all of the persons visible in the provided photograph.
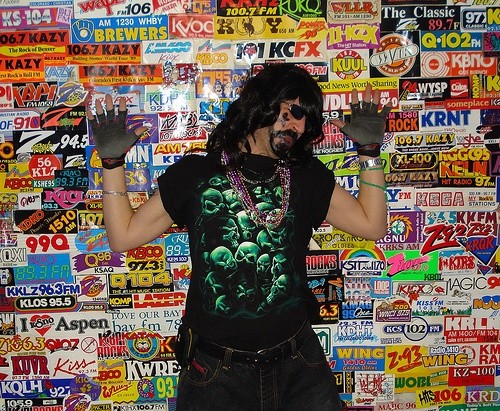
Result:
[87,63,393,411]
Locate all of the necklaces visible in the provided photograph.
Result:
[221,151,292,229]
[238,162,281,184]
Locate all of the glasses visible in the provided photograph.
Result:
[280,100,306,120]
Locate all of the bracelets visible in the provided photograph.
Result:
[360,166,388,202]
[102,189,128,196]
[359,155,383,167]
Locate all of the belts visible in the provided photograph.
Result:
[195,317,310,365]
